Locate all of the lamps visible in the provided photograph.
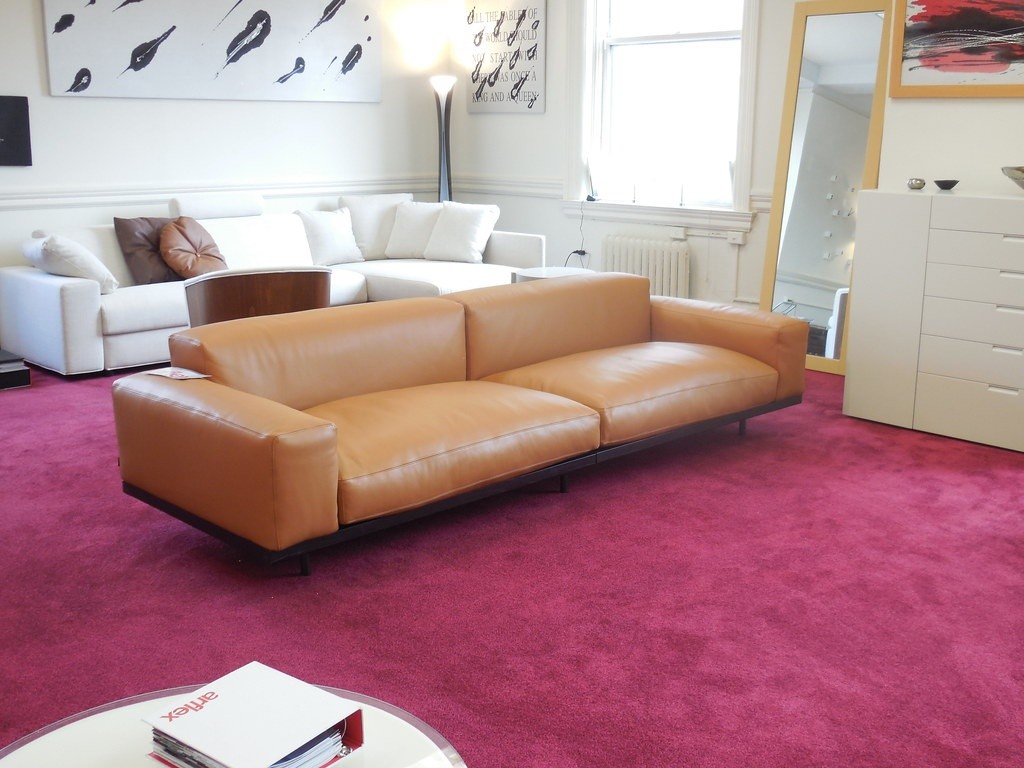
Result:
[429,74,457,199]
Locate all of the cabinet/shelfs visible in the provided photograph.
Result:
[842,190,1023,455]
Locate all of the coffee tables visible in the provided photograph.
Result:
[0,683,468,768]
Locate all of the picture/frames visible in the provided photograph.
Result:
[889,0,1024,99]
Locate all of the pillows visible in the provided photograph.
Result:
[294,209,366,266]
[384,201,444,258]
[338,193,414,261]
[426,202,501,265]
[25,235,119,293]
[159,217,229,280]
[112,216,183,285]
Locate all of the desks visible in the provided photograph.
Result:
[516,266,593,282]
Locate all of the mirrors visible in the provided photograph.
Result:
[758,0,893,376]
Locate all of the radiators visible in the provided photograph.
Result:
[599,235,689,298]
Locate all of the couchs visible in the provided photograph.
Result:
[0,217,545,381]
[111,272,810,575]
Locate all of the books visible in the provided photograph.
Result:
[141,661,365,768]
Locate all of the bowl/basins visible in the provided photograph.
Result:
[1001,166,1024,189]
[906,178,925,190]
[934,180,959,190]
